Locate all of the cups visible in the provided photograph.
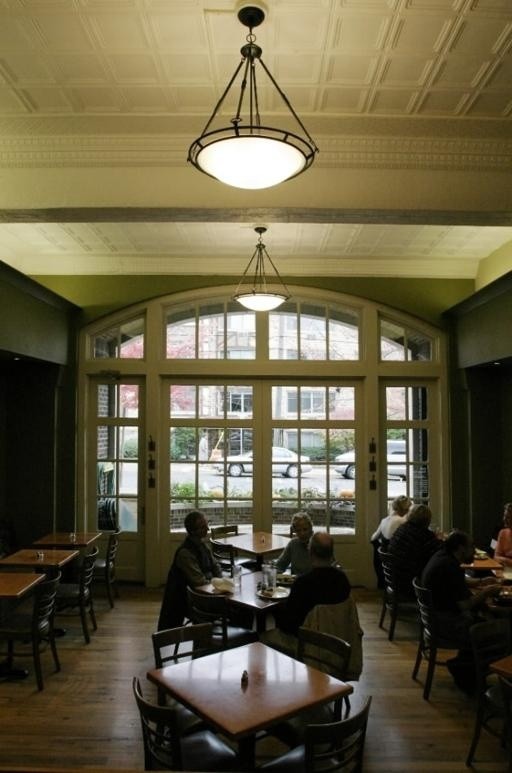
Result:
[232,564,241,589]
[262,563,277,590]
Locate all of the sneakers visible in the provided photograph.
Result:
[446,655,473,694]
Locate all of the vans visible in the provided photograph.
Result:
[334,437,408,480]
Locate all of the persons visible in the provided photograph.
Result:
[493,502,511,571]
[257,529,352,647]
[199,431,210,467]
[385,504,443,570]
[263,510,321,576]
[369,493,413,542]
[155,509,225,658]
[420,530,478,604]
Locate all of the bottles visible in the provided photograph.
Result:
[36,550,43,560]
[70,532,76,543]
[241,670,249,686]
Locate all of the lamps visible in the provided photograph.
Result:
[186,0,323,191]
[231,225,295,312]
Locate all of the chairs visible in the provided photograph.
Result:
[0,525,123,691]
[367,528,511,769]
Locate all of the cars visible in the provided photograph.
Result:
[213,446,313,476]
[220,431,252,448]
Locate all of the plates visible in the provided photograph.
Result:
[275,573,298,585]
[255,585,291,599]
